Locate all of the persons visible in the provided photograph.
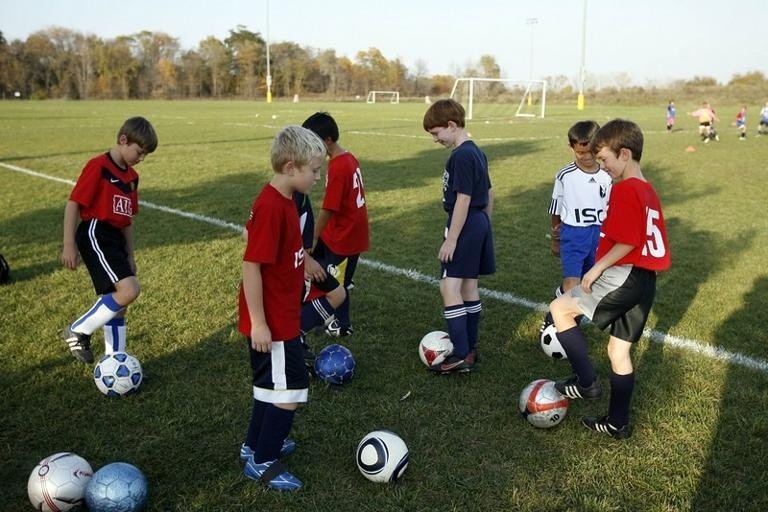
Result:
[60,118,159,365]
[550,117,671,439]
[291,190,348,374]
[422,99,496,374]
[237,125,329,494]
[539,121,603,342]
[303,113,370,336]
[758,102,768,135]
[687,103,721,143]
[666,101,677,132]
[736,105,747,139]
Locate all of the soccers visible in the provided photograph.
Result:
[28,452,92,512]
[355,429,410,486]
[419,331,452,368]
[521,379,568,427]
[313,345,355,386]
[84,463,149,512]
[93,352,142,397]
[540,323,569,361]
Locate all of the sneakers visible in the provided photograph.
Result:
[239,438,296,461]
[430,349,476,376]
[538,319,548,337]
[323,320,355,339]
[60,323,94,364]
[553,375,601,401]
[298,331,318,360]
[243,455,304,494]
[581,414,633,441]
